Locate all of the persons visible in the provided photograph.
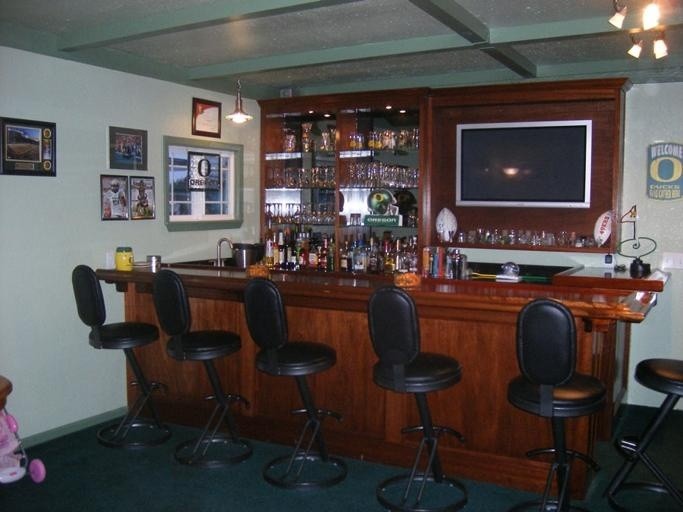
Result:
[105,179,127,219]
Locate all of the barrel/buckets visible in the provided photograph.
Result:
[233,243,266,267]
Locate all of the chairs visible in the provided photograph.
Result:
[367,286,468,512]
[244,277,348,490]
[603,358,683,512]
[72,264,173,449]
[151,270,254,466]
[506,298,606,512]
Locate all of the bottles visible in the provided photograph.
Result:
[429,246,467,279]
[115,247,160,273]
[264,122,418,274]
[436,222,595,248]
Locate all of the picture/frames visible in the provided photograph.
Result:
[108,126,149,170]
[100,174,128,220]
[129,176,156,220]
[0,117,56,177]
[191,97,222,138]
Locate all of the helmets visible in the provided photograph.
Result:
[109,179,119,190]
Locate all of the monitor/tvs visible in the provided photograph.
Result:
[455,118,592,208]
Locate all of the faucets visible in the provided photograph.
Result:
[217,237,233,267]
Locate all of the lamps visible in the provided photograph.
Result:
[225,80,253,124]
[607,0,668,59]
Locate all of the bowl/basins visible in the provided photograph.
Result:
[231,242,258,268]
